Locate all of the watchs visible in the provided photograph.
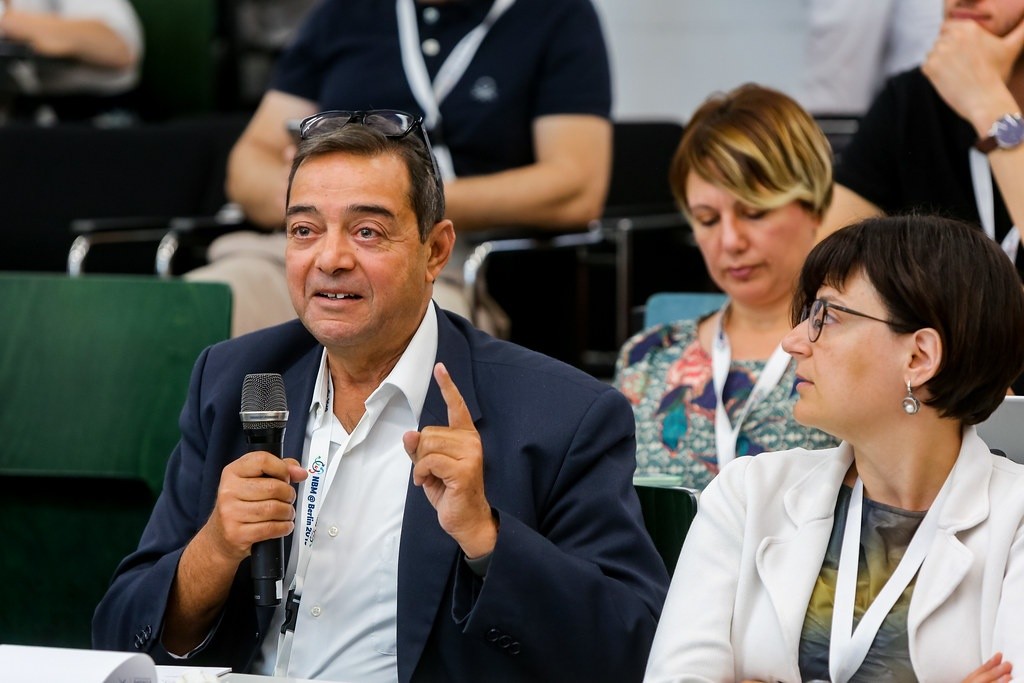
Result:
[976,112,1024,155]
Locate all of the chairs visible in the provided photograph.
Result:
[0,117,698,683]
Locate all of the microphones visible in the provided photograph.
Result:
[240,373,289,607]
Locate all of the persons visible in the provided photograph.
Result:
[0,0,613,340]
[92,113,670,682]
[611,0,1024,682]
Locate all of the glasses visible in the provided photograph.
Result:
[300,111,444,195]
[799,297,915,342]
